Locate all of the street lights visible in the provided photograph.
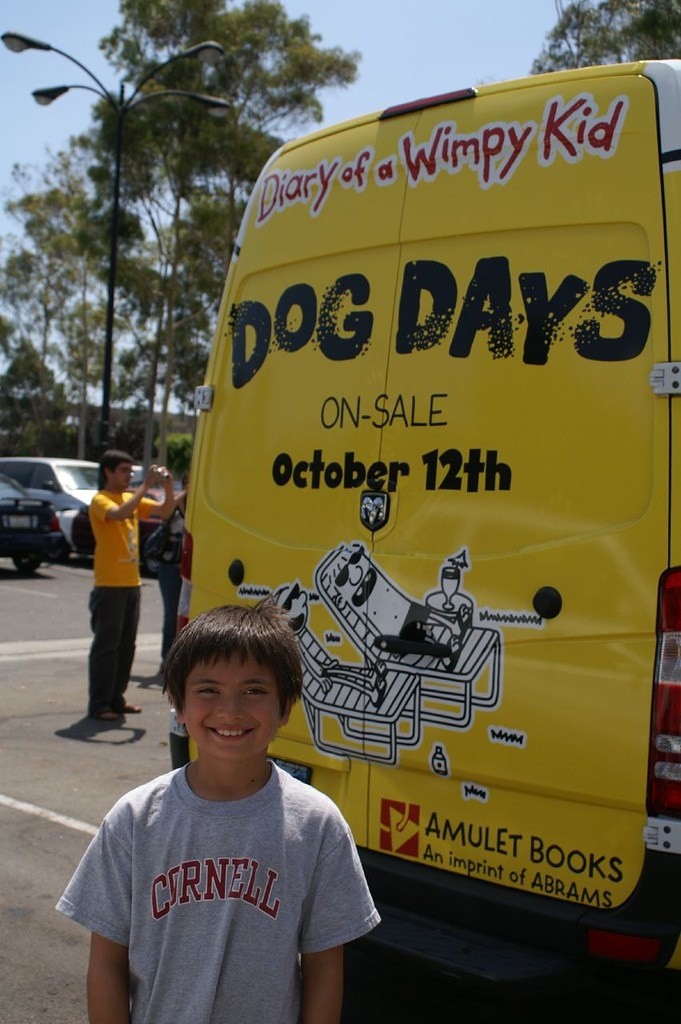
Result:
[1,30,228,465]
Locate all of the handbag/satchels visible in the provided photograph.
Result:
[144,508,179,574]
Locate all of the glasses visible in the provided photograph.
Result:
[116,465,136,477]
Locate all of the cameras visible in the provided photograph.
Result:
[156,468,170,480]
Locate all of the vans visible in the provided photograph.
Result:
[169,61,681,1024]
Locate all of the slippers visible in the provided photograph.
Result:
[112,703,141,713]
[89,711,118,720]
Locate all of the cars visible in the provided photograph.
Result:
[0,455,183,579]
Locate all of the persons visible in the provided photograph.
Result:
[88,450,176,718]
[54,599,380,1024]
[158,470,189,672]
